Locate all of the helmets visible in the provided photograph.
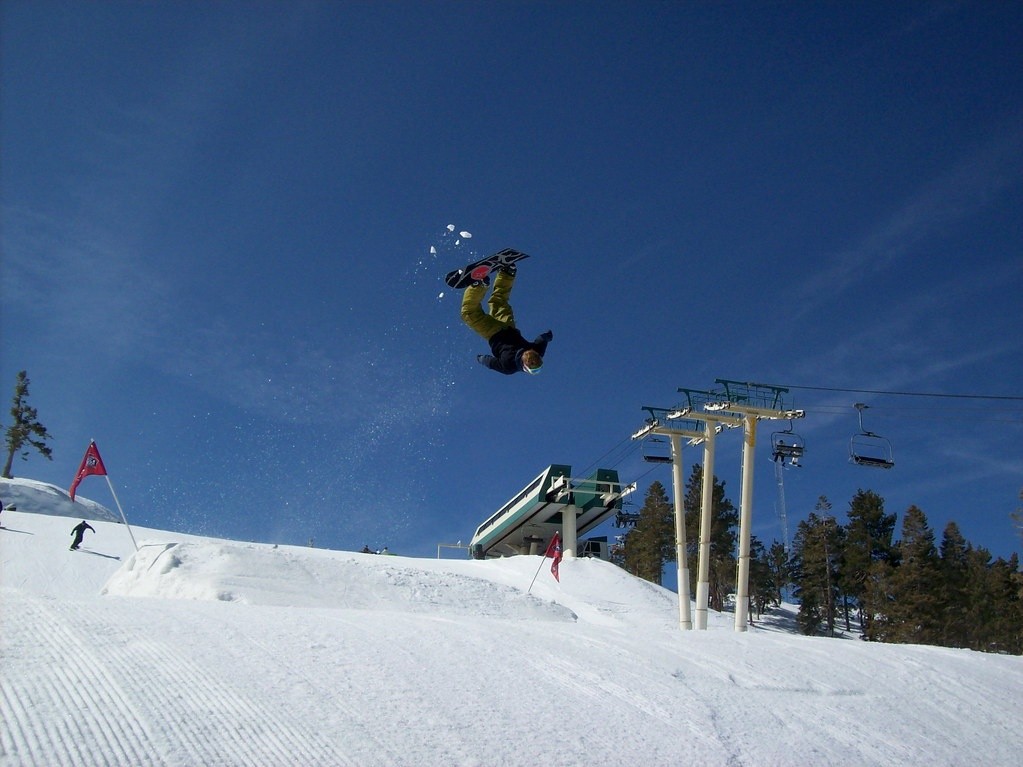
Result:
[522,363,544,376]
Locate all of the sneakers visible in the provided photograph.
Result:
[480,276,491,286]
[503,262,517,275]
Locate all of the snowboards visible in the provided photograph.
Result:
[445,247,530,289]
[69,546,79,551]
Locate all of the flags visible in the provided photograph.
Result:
[546,532,562,583]
[69,441,107,501]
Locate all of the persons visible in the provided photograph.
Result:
[361,543,390,555]
[461,262,553,375]
[68,520,95,550]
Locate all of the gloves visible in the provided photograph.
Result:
[546,329,553,340]
[476,354,483,363]
